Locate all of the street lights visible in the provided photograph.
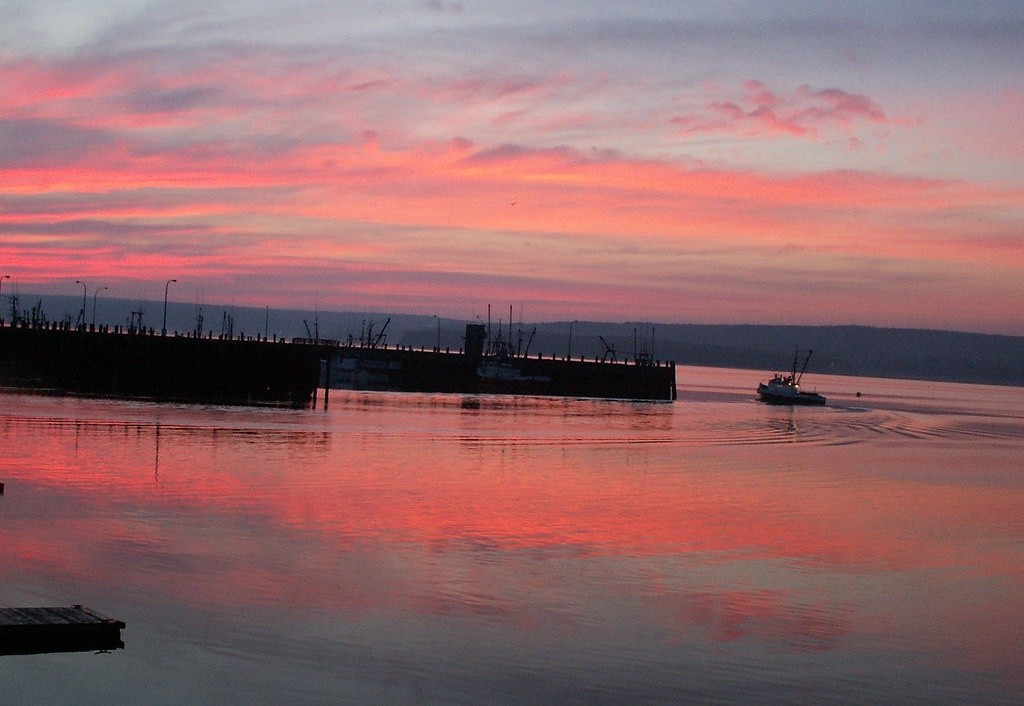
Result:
[567,320,579,356]
[76,280,87,324]
[93,287,109,325]
[265,305,269,337]
[434,314,440,352]
[0,274,11,294]
[163,279,177,330]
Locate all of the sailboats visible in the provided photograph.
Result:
[756,343,827,408]
